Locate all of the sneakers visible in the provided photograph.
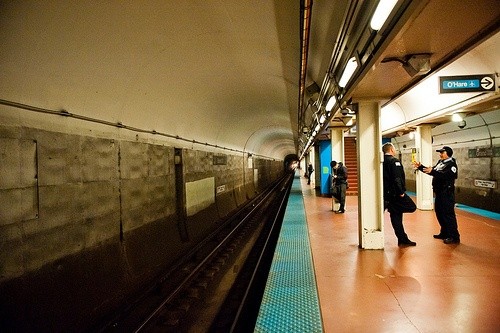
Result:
[433,232,448,239]
[444,237,460,243]
[384,201,388,211]
[398,237,416,246]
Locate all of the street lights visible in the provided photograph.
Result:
[452,109,493,201]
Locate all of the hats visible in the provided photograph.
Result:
[436,146,453,157]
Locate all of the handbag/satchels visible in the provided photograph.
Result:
[346,182,349,189]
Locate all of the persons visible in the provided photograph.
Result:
[412,146,460,244]
[382,142,416,246]
[307,165,312,185]
[330,161,348,214]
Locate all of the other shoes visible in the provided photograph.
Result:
[337,209,344,213]
